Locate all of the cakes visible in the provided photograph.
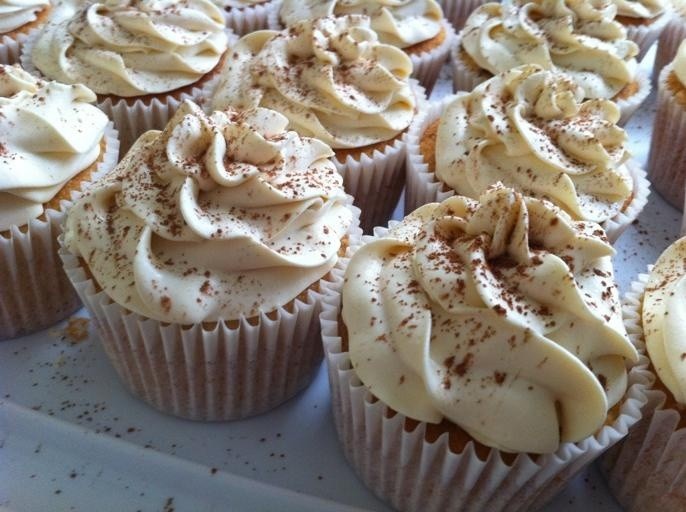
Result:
[339,180,640,511]
[0,0,50,62]
[65,99,353,328]
[593,235,685,512]
[212,0,498,99]
[405,63,636,246]
[451,0,685,210]
[0,65,110,341]
[32,0,233,156]
[212,14,419,235]
